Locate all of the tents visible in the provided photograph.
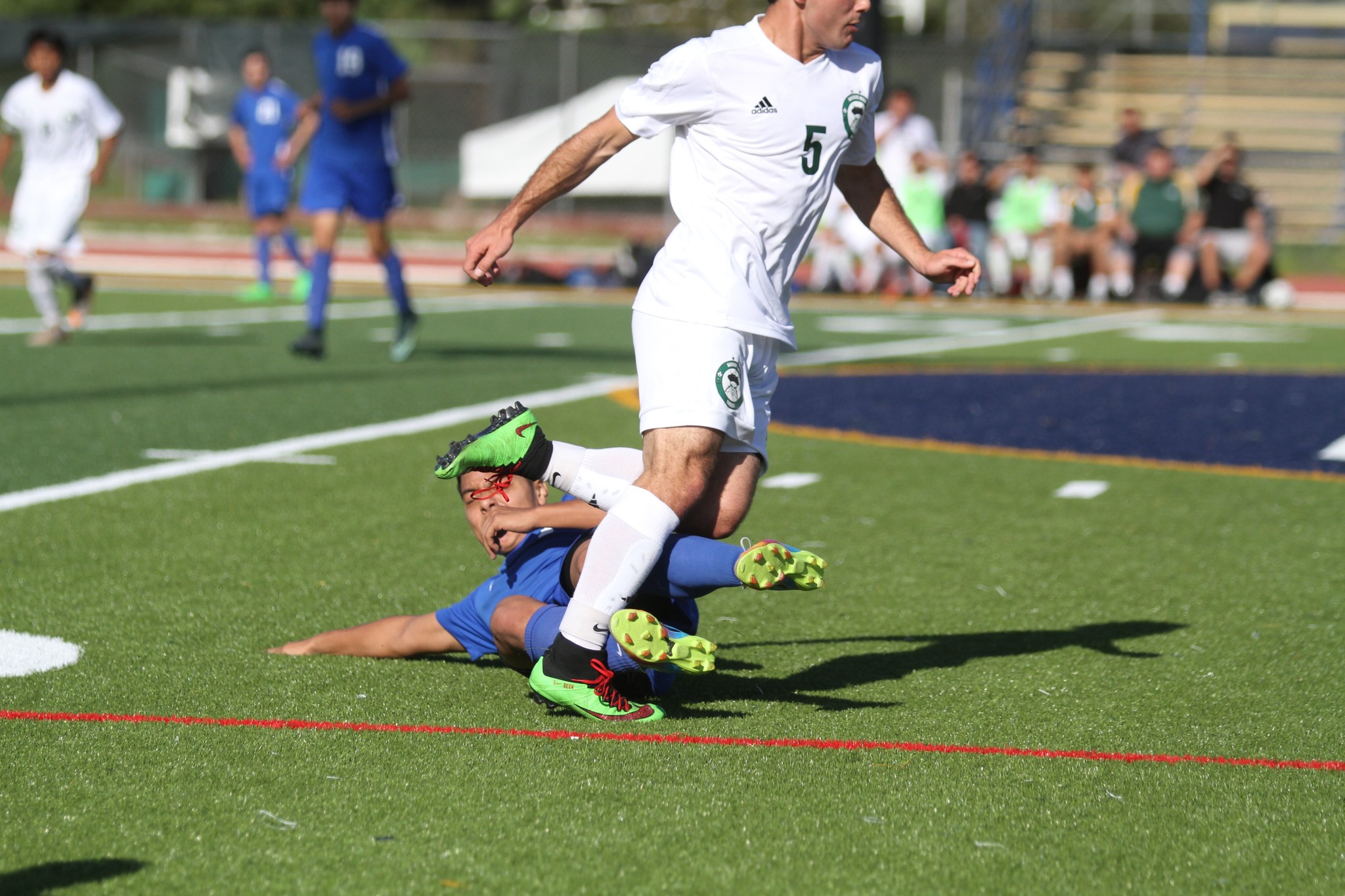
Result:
[459,76,675,197]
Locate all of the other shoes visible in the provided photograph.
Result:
[289,269,316,304]
[290,329,324,358]
[391,317,424,361]
[240,281,271,302]
[66,274,92,329]
[29,326,67,347]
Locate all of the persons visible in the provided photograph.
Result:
[810,84,1297,307]
[266,470,828,699]
[0,28,126,347]
[226,48,323,304]
[464,0,981,722]
[286,0,422,361]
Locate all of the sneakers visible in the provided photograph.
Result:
[607,608,718,677]
[733,537,827,592]
[434,400,546,502]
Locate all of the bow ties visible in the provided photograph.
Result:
[524,650,667,724]
